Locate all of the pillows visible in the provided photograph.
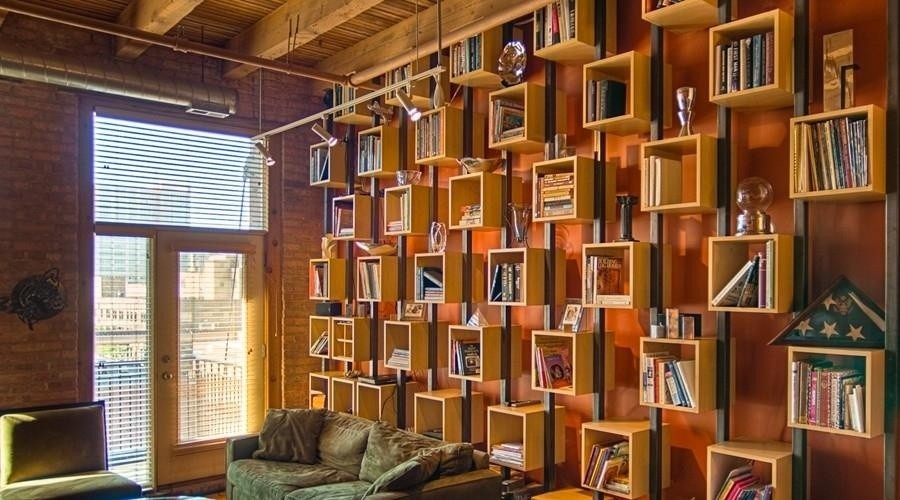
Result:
[315,411,375,476]
[358,422,448,484]
[252,409,326,465]
[359,447,441,500]
[410,442,473,476]
[0,404,107,484]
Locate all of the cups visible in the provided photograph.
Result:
[429,222,447,253]
[683,317,694,339]
[554,133,568,158]
[651,325,664,337]
[320,236,339,259]
[359,305,369,316]
[674,86,699,135]
[666,308,680,338]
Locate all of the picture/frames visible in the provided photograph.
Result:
[557,298,584,333]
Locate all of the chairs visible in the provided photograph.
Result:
[0,399,142,499]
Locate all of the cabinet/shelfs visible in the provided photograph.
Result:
[308,0,899,500]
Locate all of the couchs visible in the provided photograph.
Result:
[224,409,501,500]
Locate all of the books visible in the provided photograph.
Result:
[451,337,480,375]
[644,156,682,207]
[311,330,328,354]
[387,347,409,368]
[645,1,681,13]
[358,374,412,385]
[491,441,523,468]
[534,343,572,388]
[312,262,328,297]
[791,358,865,433]
[586,254,631,305]
[312,0,577,238]
[586,79,626,123]
[583,439,630,495]
[715,31,774,96]
[417,266,443,300]
[794,116,868,193]
[489,263,524,302]
[359,261,380,299]
[643,352,695,409]
[716,465,772,499]
[712,239,774,309]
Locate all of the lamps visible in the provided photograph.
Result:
[251,0,446,166]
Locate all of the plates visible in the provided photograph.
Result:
[500,42,528,85]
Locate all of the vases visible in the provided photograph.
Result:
[676,87,696,137]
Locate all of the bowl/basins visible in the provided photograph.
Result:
[457,157,503,173]
[354,241,397,256]
[396,169,422,185]
